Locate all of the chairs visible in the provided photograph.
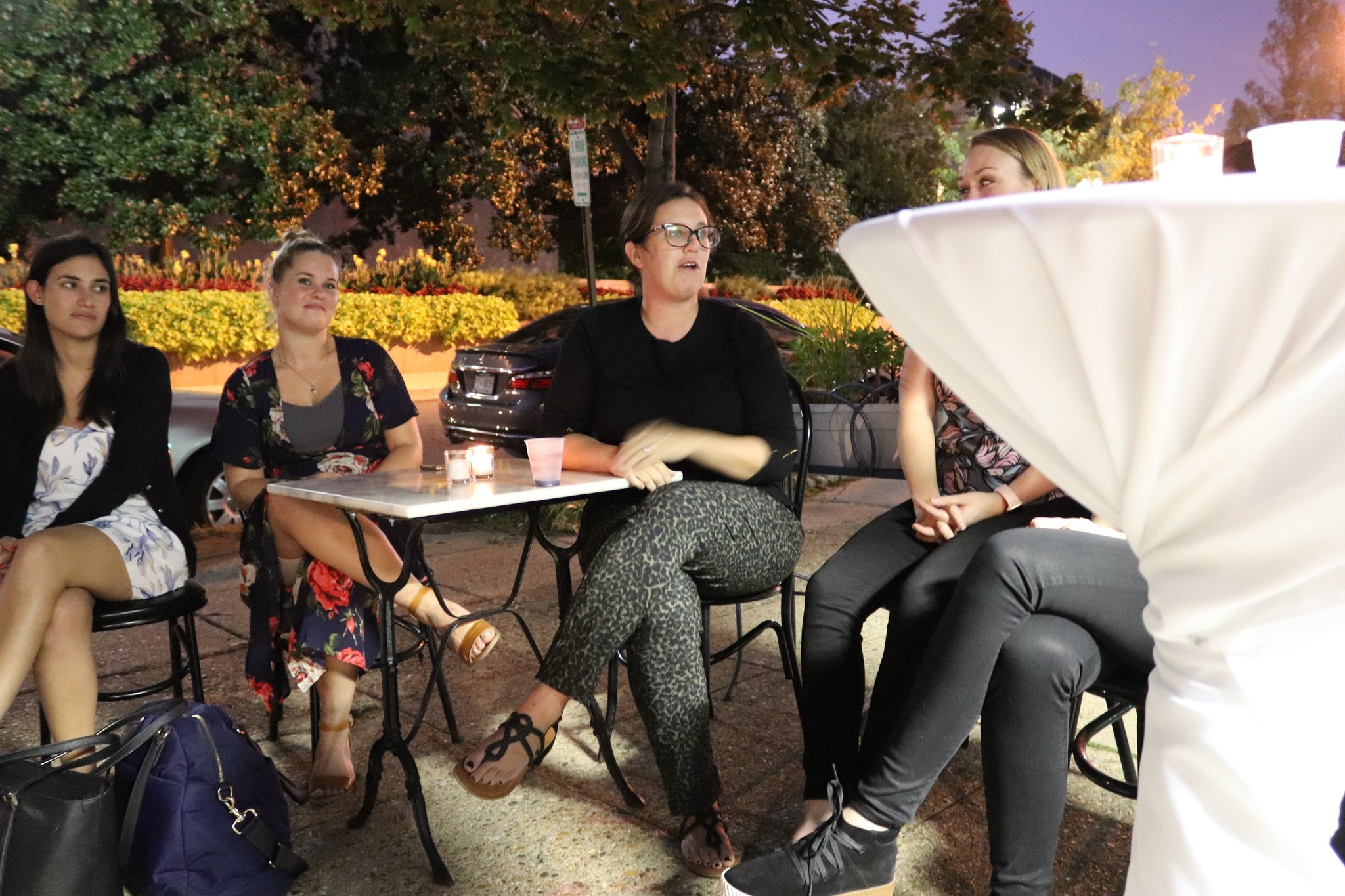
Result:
[37,361,1148,801]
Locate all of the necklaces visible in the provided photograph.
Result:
[277,333,329,393]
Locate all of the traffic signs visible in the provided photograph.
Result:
[569,131,592,206]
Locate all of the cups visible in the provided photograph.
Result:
[467,445,494,479]
[444,449,471,483]
[524,438,565,488]
[1150,134,1223,180]
[1247,120,1344,173]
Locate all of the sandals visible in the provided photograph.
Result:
[678,797,739,879]
[409,584,501,670]
[308,712,354,806]
[453,710,564,800]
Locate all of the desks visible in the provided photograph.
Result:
[834,165,1345,896]
[265,446,684,888]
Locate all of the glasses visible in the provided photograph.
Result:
[636,223,722,250]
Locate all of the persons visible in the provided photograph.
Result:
[210,228,501,785]
[793,128,1092,845]
[727,517,1155,896]
[0,233,196,780]
[456,184,800,878]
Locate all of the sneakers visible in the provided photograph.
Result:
[720,764,903,896]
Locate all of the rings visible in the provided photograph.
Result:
[643,448,649,453]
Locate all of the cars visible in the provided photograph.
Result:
[0,327,245,530]
[439,298,897,459]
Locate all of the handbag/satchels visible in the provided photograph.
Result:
[113,696,310,896]
[0,697,190,896]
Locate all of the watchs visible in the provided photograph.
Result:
[994,483,1023,511]
